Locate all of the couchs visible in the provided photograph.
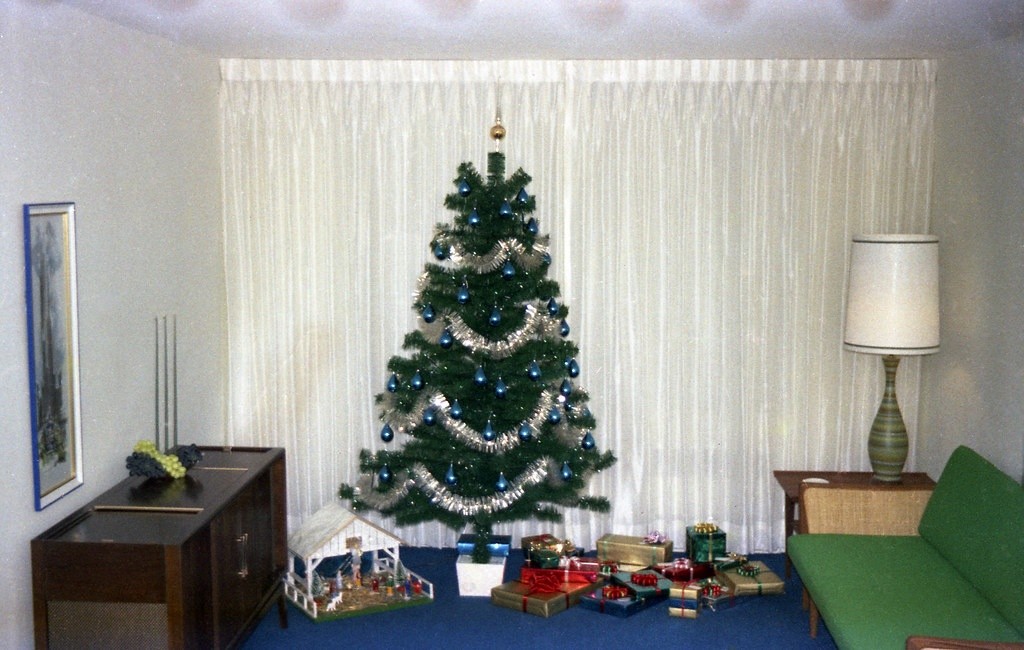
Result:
[787,443,1024,650]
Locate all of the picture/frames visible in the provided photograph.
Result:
[23,201,86,510]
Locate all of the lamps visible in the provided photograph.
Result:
[844,233,940,481]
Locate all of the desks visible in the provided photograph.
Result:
[774,469,938,612]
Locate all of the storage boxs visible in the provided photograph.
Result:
[457,533,511,557]
[491,525,786,618]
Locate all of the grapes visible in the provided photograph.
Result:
[125,439,202,479]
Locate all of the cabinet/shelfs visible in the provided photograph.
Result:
[31,445,288,650]
